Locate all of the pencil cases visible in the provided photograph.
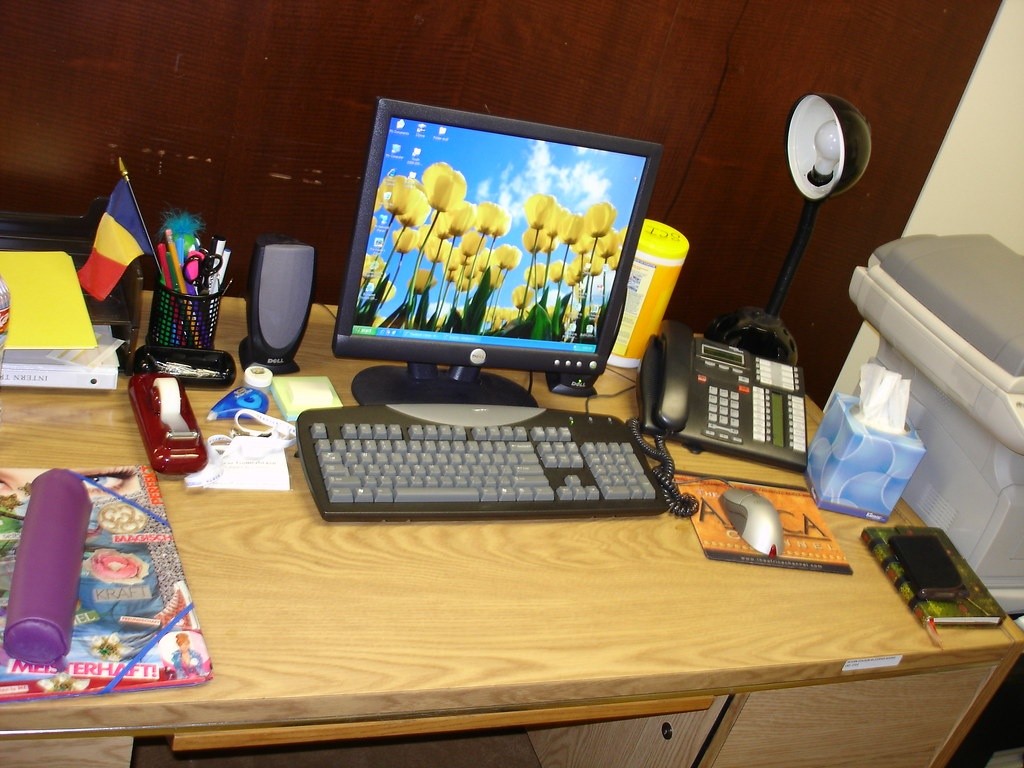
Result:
[4,467,93,663]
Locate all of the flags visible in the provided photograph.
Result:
[77,180,153,301]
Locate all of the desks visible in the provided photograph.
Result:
[1,290,1024,768]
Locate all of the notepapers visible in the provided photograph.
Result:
[270,376,343,421]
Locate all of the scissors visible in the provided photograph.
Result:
[182,254,223,333]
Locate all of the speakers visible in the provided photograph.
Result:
[545,288,627,397]
[239,232,318,374]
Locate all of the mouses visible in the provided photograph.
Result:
[719,488,785,558]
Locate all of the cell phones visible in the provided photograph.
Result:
[889,534,969,601]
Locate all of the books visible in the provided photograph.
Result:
[0,465,215,702]
[861,527,1006,629]
[0,325,119,390]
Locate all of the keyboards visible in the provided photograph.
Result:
[296,404,671,523]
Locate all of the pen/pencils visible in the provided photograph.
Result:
[153,228,231,350]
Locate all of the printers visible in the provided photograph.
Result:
[830,233,1024,616]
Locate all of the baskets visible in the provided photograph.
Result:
[145,279,221,351]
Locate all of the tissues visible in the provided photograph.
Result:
[804,360,929,525]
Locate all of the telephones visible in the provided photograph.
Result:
[636,320,808,472]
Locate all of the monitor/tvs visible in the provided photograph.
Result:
[332,97,662,409]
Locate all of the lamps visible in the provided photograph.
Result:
[704,94,872,367]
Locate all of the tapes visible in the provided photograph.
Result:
[152,377,190,433]
[244,366,274,388]
